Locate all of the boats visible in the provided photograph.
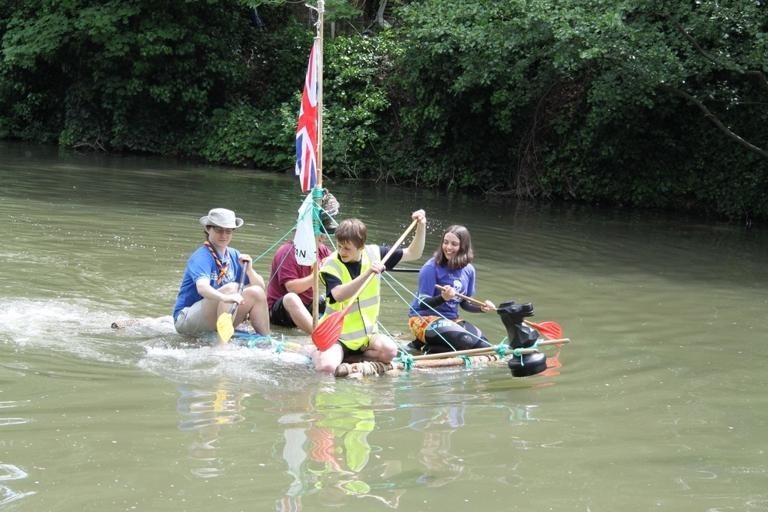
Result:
[110,317,571,378]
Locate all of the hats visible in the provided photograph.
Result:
[199,208,244,229]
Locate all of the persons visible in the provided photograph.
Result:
[170,206,279,351]
[303,208,428,374]
[267,220,332,334]
[406,223,498,355]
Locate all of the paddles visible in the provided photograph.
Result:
[215,260,248,345]
[432,283,564,350]
[311,217,419,352]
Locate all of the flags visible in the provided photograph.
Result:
[293,36,321,193]
